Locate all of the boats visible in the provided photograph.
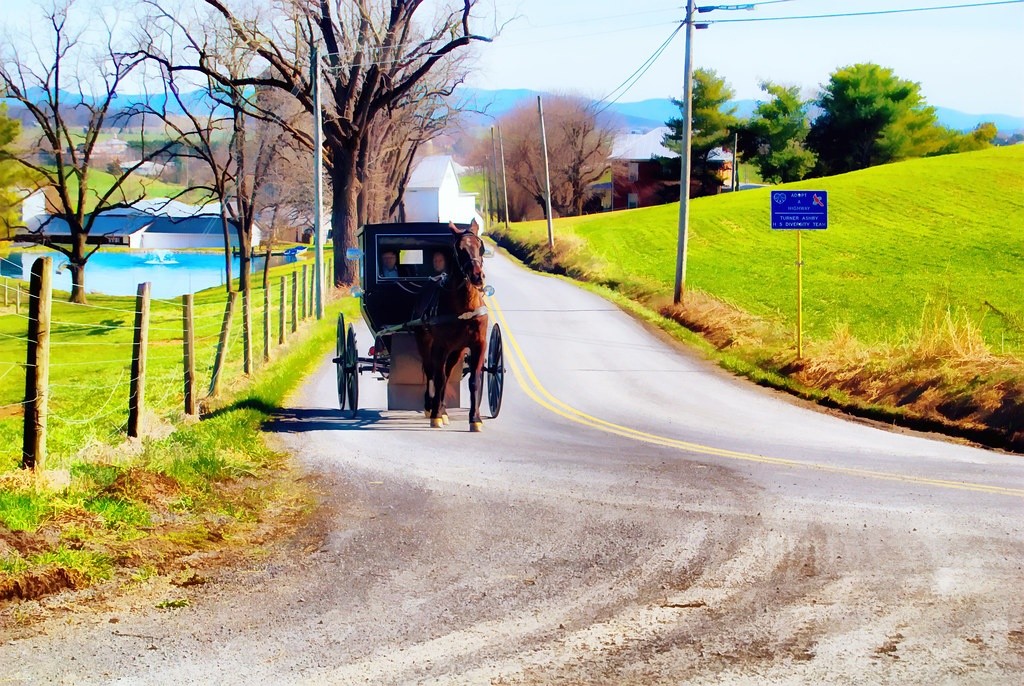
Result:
[285,245,308,254]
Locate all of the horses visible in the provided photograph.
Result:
[409,217,488,434]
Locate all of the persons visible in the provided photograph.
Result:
[431,251,448,277]
[379,249,408,277]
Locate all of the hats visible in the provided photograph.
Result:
[381,247,400,255]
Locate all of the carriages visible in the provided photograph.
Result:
[332,221,506,432]
